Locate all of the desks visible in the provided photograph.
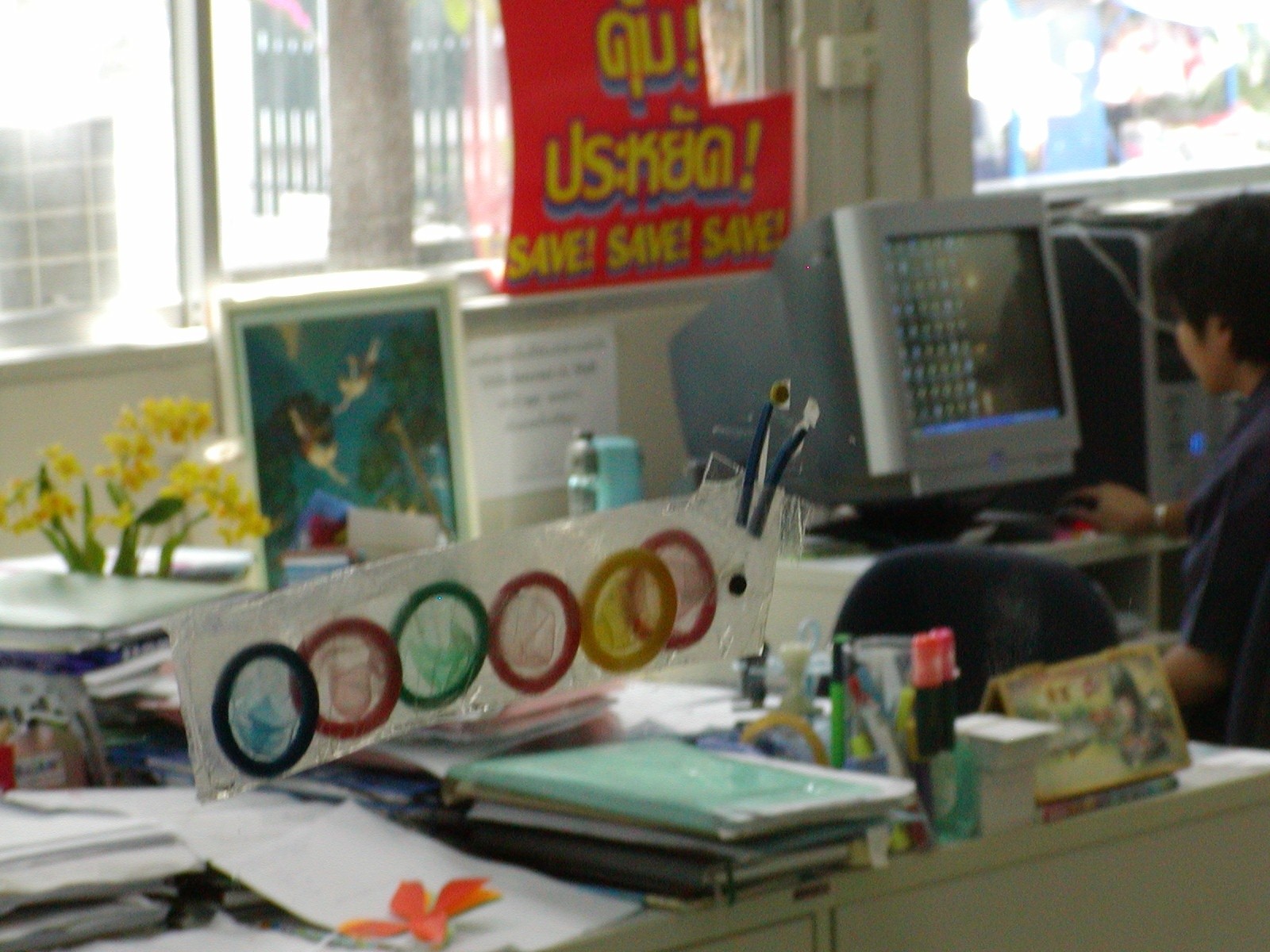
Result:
[775,526,1189,660]
[532,740,1270,952]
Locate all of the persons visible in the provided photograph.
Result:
[1060,190,1270,752]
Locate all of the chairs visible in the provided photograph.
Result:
[836,540,1116,731]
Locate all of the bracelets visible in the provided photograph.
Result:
[1152,500,1172,530]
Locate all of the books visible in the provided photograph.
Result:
[0,692,919,952]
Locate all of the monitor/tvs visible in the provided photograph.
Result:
[669,191,1084,563]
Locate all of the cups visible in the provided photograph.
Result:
[570,431,648,519]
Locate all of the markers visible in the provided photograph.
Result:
[912,626,955,758]
[849,677,926,853]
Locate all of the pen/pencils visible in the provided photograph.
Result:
[830,634,850,770]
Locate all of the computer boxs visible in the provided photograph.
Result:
[1044,211,1240,516]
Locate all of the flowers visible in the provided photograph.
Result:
[0,391,288,580]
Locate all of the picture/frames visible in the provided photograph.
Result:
[205,268,481,591]
